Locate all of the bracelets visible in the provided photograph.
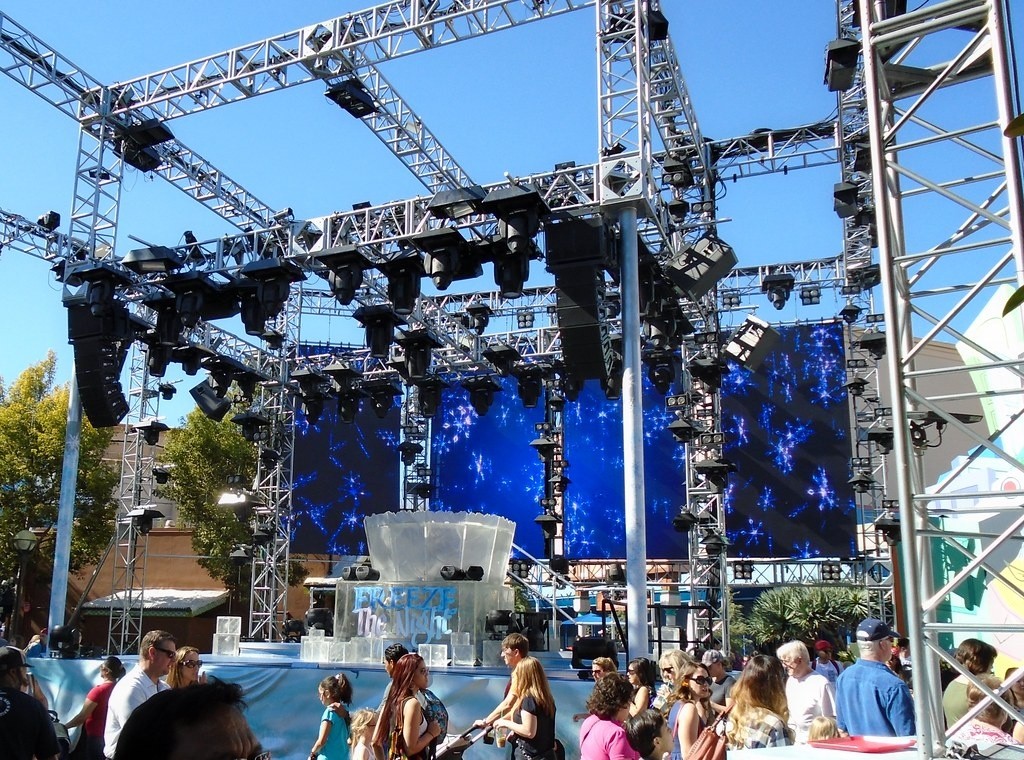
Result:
[428,732,434,738]
[482,718,486,724]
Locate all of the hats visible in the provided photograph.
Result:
[0,646,35,668]
[40,628,48,635]
[856,617,901,641]
[701,648,724,667]
[815,640,834,650]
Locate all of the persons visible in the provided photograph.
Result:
[307,643,441,760]
[473,633,556,760]
[26,628,47,658]
[0,645,62,760]
[63,630,271,760]
[575,619,1024,760]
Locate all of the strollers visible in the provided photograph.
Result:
[430,722,490,760]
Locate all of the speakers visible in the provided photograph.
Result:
[723,316,779,373]
[666,235,737,302]
[545,214,614,383]
[68,304,129,427]
[440,565,484,580]
[189,379,231,423]
[342,567,381,581]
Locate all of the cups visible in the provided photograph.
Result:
[652,684,673,709]
[495,726,507,748]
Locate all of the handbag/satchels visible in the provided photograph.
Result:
[683,703,735,760]
[552,739,565,760]
[388,696,436,760]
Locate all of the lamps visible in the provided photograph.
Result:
[37,0,1024,581]
[483,608,551,653]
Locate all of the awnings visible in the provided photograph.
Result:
[80,588,228,618]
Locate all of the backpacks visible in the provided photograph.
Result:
[422,688,449,745]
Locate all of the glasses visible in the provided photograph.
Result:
[418,668,430,675]
[234,751,270,760]
[823,649,831,653]
[179,660,203,668]
[591,670,607,674]
[690,676,713,685]
[100,664,112,673]
[662,667,673,673]
[155,647,176,658]
[627,670,635,675]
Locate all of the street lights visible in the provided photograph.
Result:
[7,530,40,644]
